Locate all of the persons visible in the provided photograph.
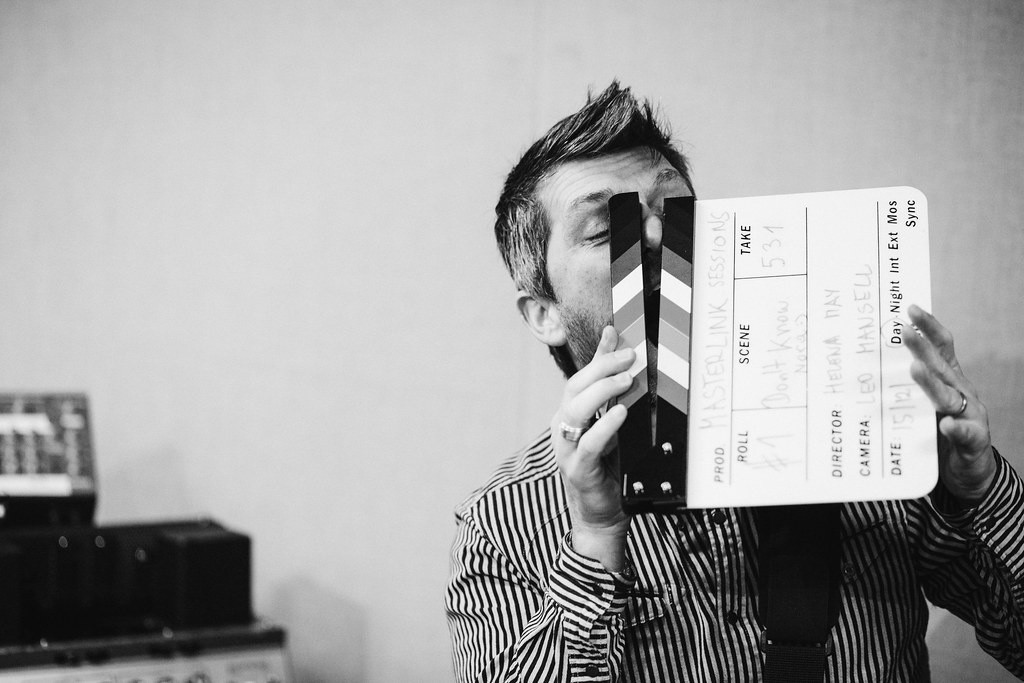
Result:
[445,81,1023,682]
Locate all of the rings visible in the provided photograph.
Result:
[954,391,967,418]
[559,424,587,445]
